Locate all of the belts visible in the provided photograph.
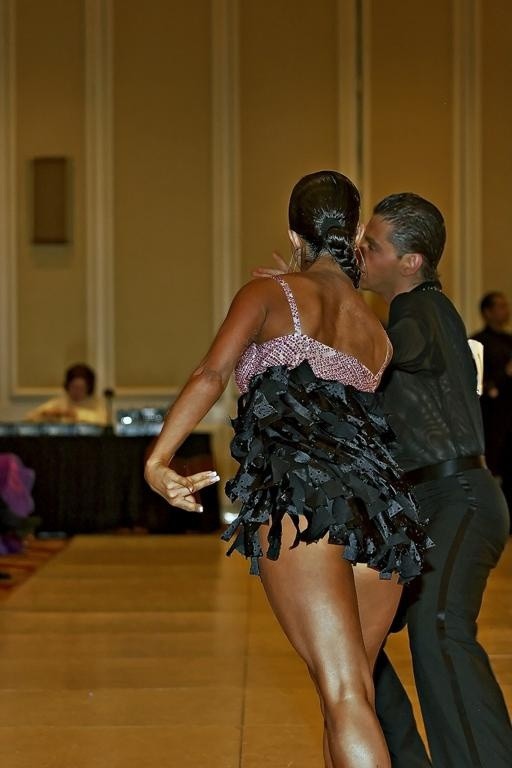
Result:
[402,454,487,485]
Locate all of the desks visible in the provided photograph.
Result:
[1,433,221,536]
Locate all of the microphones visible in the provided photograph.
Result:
[102,386,119,434]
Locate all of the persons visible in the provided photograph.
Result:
[468,289,511,536]
[26,361,116,427]
[355,191,511,767]
[141,168,435,767]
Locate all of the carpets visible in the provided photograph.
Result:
[0,539,69,602]
